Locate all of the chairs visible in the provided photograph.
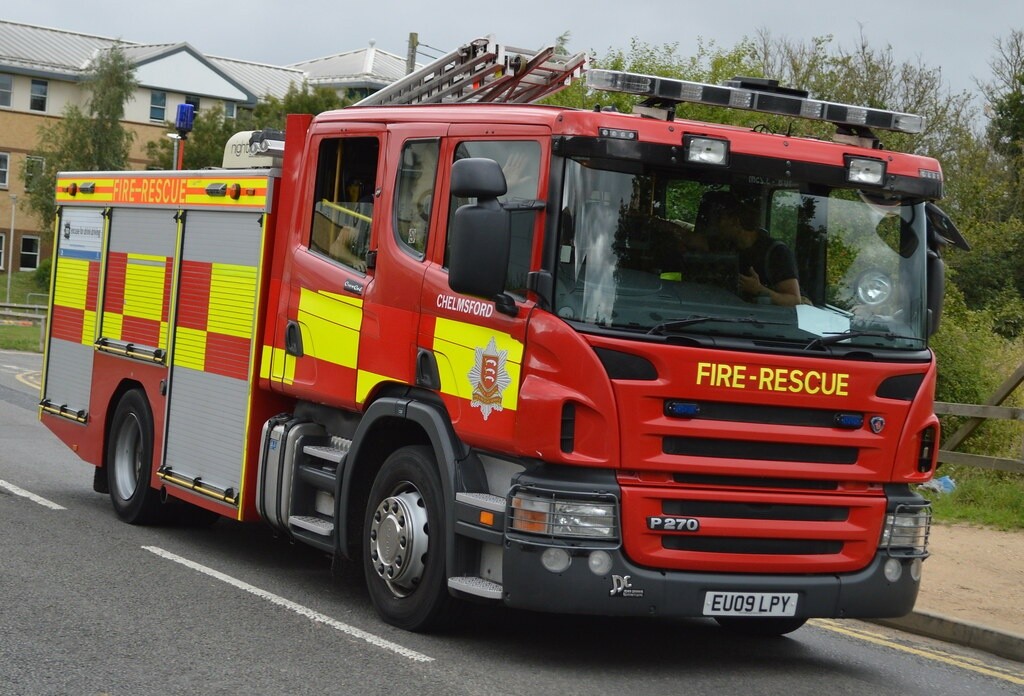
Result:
[554,191,748,259]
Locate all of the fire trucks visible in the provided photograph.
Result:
[38,33,972,636]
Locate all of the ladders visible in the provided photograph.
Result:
[348,40,586,105]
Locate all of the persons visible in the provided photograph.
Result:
[500,153,592,265]
[685,194,801,305]
[330,177,413,272]
